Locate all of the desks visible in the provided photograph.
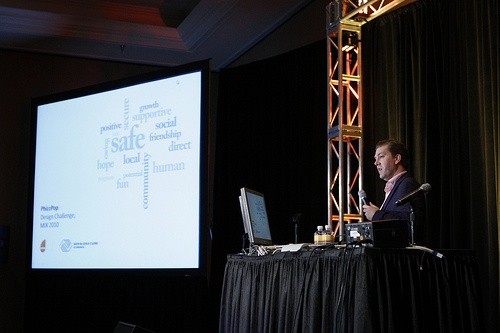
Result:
[218,248,480,333]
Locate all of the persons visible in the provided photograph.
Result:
[361,137,427,247]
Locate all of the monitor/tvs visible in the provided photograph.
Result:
[238,187,273,254]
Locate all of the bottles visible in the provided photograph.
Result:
[322,225,334,245]
[314,226,323,245]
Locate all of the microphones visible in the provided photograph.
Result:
[358,190,370,206]
[394,183,432,207]
